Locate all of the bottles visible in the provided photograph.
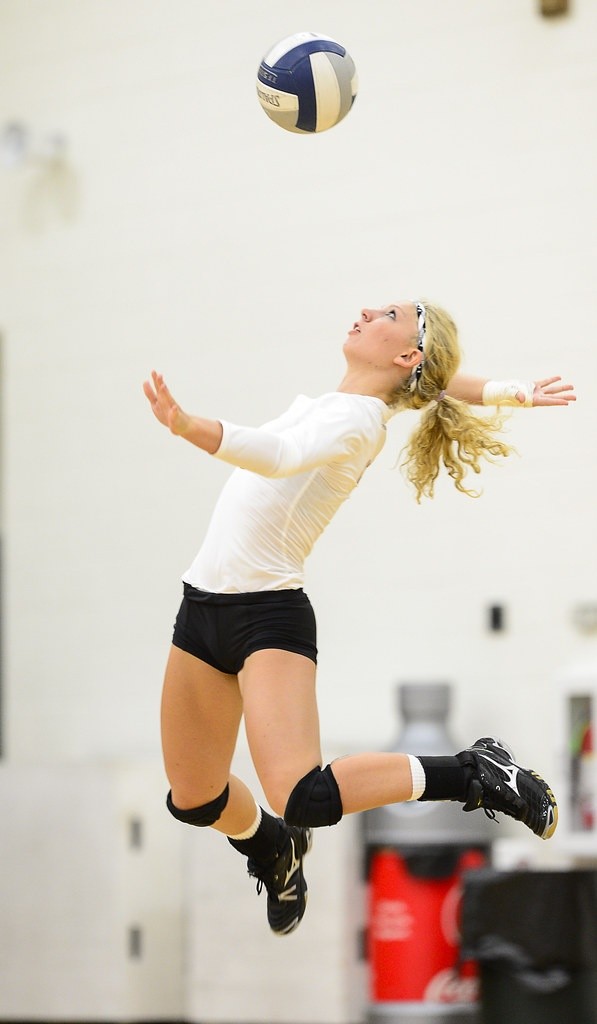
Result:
[360,681,512,1024]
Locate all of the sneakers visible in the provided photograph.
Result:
[247,818,312,934]
[457,735,558,840]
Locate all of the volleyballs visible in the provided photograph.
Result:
[256,33,358,136]
